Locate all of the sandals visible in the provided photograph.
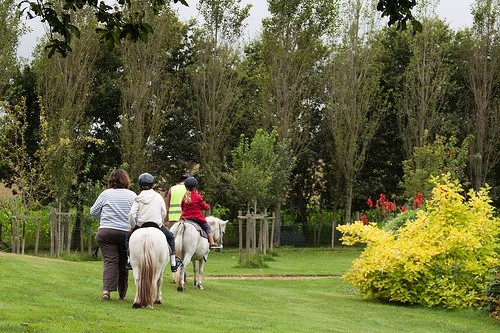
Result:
[102,293,110,300]
[119,298,127,301]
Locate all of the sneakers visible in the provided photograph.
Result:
[210,243,219,247]
[171,260,180,272]
[126,263,132,270]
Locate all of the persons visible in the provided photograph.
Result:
[126,173,179,272]
[164,175,199,282]
[179,176,222,249]
[89,170,137,300]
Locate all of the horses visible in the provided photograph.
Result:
[170,216,229,292]
[128,227,169,309]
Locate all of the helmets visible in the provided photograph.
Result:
[184,177,198,185]
[138,173,154,183]
[179,173,190,179]
